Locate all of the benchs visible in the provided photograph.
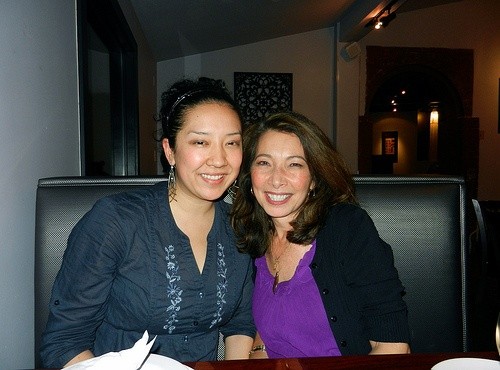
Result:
[35,177,468,369]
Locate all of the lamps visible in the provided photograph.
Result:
[374,8,397,30]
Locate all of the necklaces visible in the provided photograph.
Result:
[270,237,291,295]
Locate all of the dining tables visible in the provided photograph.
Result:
[181,351,500,370]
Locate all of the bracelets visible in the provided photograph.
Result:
[249,344,266,355]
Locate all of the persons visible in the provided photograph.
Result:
[232,112,411,359]
[38,77,256,370]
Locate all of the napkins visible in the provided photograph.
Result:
[76,328,158,370]
